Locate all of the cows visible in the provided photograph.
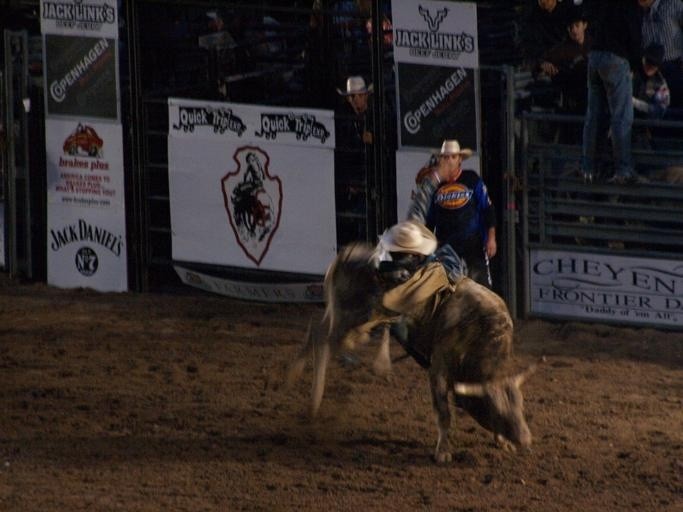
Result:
[271,244,549,464]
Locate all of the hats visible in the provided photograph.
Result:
[644,41,665,65]
[380,219,438,256]
[432,140,473,159]
[336,76,374,96]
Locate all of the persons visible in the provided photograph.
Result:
[364,139,497,290]
[533,1,683,183]
[336,77,373,147]
